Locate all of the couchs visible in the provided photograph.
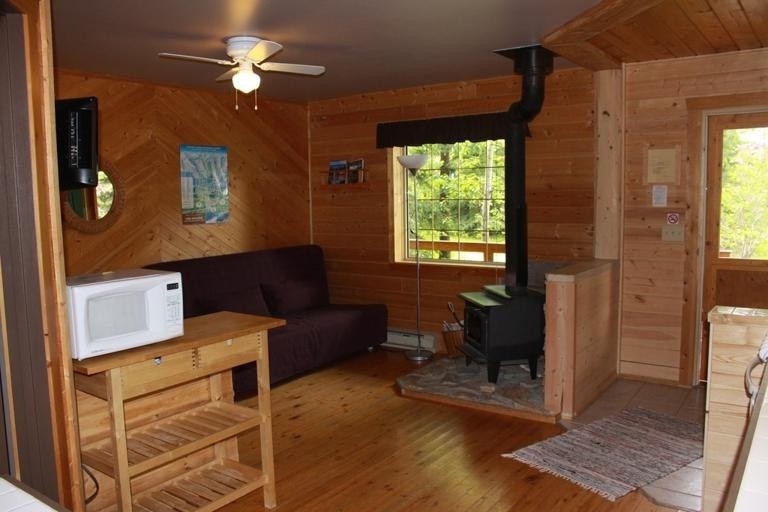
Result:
[142,245,390,401]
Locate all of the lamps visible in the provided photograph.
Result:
[397,153,431,362]
[232,68,261,94]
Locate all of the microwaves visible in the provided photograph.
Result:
[66,266,185,360]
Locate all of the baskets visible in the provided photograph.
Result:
[440,319,468,360]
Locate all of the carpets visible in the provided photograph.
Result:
[501,405,704,503]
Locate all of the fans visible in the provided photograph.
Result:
[160,35,326,81]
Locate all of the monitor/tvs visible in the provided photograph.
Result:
[55,96,98,190]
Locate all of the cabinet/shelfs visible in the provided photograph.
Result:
[69,310,286,512]
